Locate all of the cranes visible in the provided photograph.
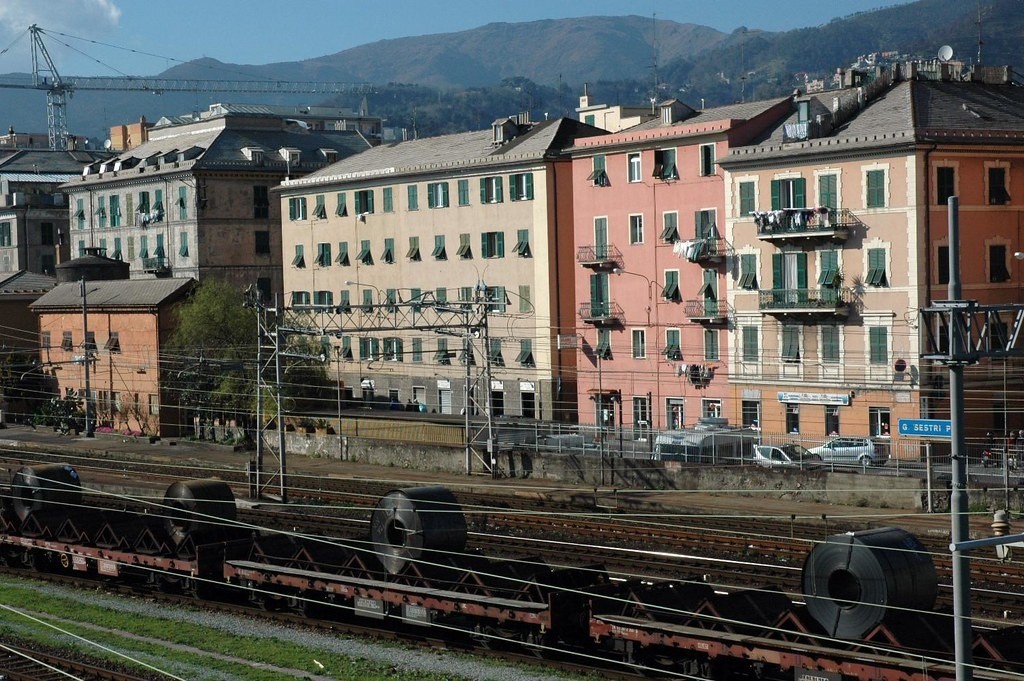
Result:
[0,24,376,150]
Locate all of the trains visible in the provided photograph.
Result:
[0,520,1024,681]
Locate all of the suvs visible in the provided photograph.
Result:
[753,446,821,472]
[808,436,889,467]
